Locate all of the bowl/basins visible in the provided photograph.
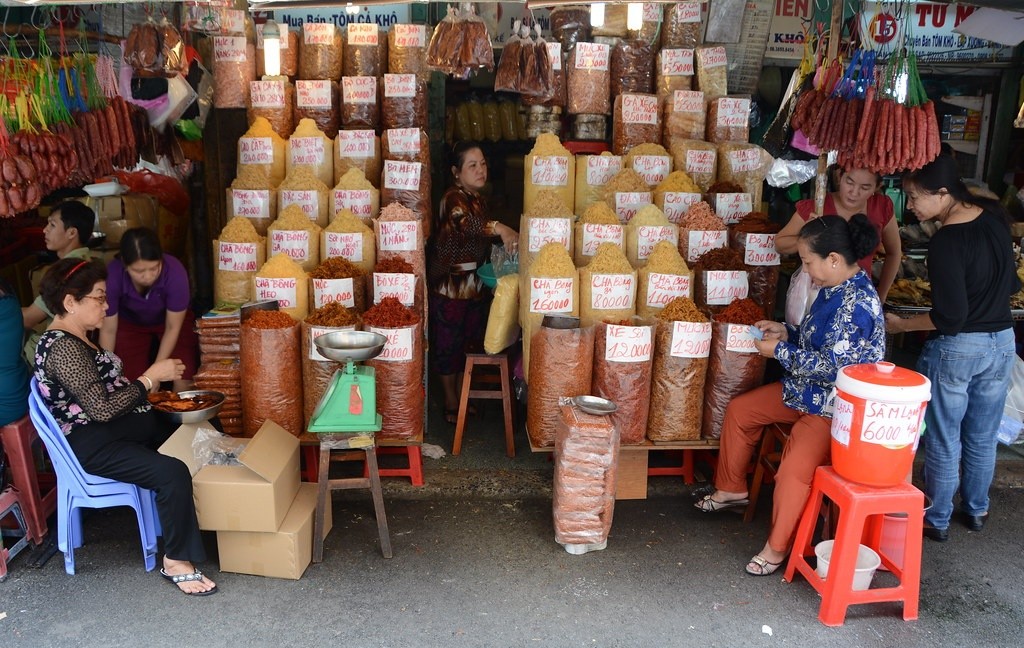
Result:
[82,182,123,197]
[573,395,618,416]
[89,231,107,248]
[477,262,518,288]
[158,390,226,424]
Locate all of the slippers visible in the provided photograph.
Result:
[696,496,749,513]
[448,409,459,424]
[160,567,217,596]
[745,553,783,575]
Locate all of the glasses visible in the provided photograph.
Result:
[79,295,107,304]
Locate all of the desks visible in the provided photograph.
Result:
[884,308,936,364]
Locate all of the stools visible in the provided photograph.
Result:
[313,432,393,562]
[0,415,57,582]
[452,345,515,457]
[742,423,924,625]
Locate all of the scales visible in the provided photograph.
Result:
[306,330,387,431]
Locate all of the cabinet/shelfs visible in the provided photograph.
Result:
[940,94,992,184]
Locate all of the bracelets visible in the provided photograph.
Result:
[141,374,152,390]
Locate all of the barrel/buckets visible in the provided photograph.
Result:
[877,494,934,571]
[824,362,932,489]
[813,540,881,598]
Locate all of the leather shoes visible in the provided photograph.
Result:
[966,511,990,531]
[924,521,948,543]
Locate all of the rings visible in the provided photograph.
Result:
[512,243,518,246]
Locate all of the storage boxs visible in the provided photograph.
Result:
[216,482,332,579]
[99,195,185,255]
[157,420,301,530]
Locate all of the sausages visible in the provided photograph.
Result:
[790,85,941,176]
[0,95,141,220]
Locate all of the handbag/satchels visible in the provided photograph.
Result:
[784,266,820,325]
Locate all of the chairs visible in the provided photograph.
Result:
[28,374,162,576]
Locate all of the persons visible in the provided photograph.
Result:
[694,213,886,575]
[774,166,901,306]
[34,257,217,596]
[0,199,95,432]
[98,228,199,393]
[903,151,1021,541]
[429,139,518,424]
